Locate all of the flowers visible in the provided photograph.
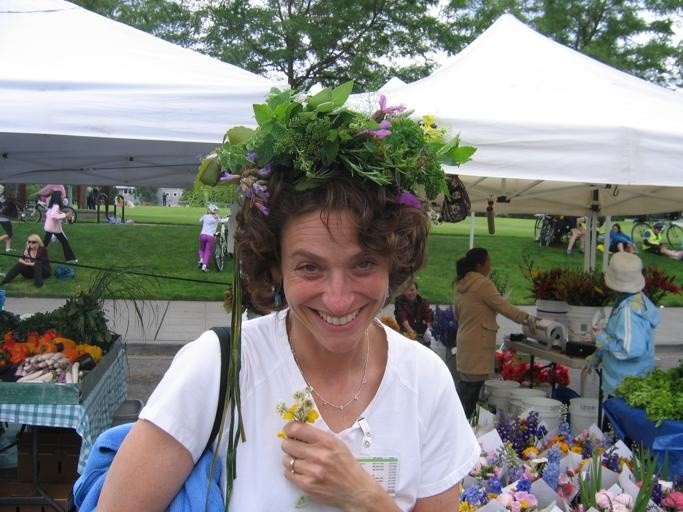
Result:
[532,267,682,305]
[190,85,470,218]
[458,405,682,511]
[496,351,572,386]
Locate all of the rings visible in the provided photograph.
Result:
[289,458,296,473]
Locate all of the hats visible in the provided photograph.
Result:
[605,252,645,294]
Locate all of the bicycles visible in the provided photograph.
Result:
[630,214,683,252]
[214,214,234,272]
[22,197,78,224]
[534,214,576,247]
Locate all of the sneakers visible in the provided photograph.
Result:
[202,266,208,273]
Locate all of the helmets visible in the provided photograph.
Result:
[207,205,219,214]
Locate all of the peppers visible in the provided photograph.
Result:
[0,328,102,367]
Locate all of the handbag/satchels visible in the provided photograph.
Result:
[73,422,225,512]
[56,266,74,280]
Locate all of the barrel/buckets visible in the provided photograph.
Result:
[536,298,571,334]
[568,396,606,433]
[481,376,564,433]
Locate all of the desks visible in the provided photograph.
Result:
[503,334,662,399]
[0,338,130,511]
[602,397,683,480]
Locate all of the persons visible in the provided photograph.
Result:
[641,223,683,261]
[579,251,663,451]
[93,78,481,512]
[0,233,52,288]
[161,191,167,206]
[0,184,17,253]
[609,222,637,254]
[393,281,432,349]
[197,204,230,272]
[565,216,586,256]
[43,191,79,264]
[450,246,543,423]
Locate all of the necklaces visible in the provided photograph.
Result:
[287,326,370,411]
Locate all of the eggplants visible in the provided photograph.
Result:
[14,352,83,384]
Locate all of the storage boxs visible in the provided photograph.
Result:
[14,425,80,483]
[1,481,73,512]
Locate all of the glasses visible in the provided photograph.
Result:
[28,240,37,244]
